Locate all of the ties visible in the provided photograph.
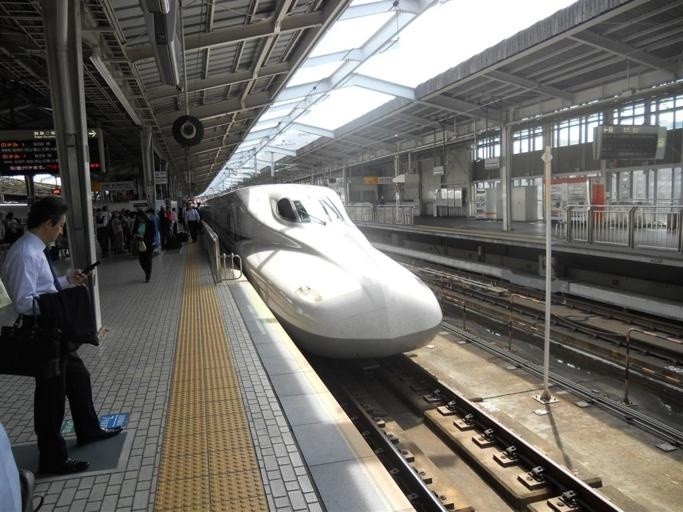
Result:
[44,247,63,291]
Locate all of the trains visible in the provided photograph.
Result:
[0,203,28,222]
[198,181,444,364]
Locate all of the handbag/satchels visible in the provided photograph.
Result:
[0,297,62,379]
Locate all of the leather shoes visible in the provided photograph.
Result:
[78,426,123,446]
[39,456,89,474]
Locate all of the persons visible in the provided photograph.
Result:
[0,199,202,282]
[379,195,385,208]
[1,196,123,473]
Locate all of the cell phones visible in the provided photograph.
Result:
[79,260,101,277]
